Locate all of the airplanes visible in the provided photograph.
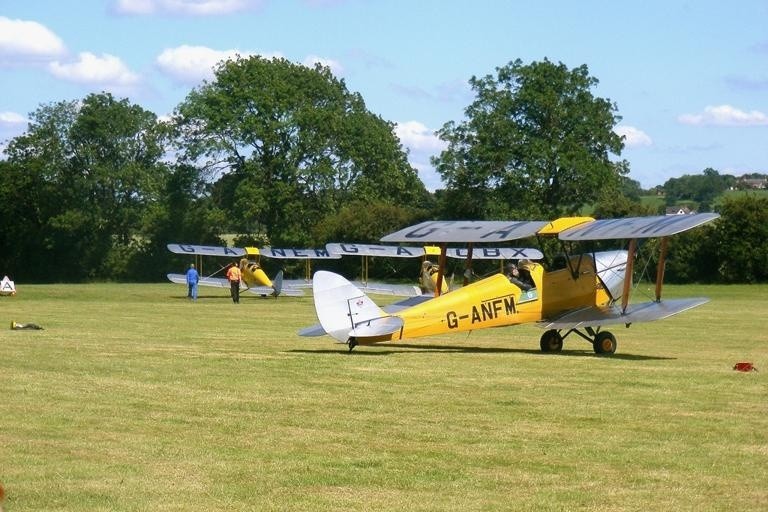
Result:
[164,241,343,301]
[323,240,544,303]
[295,211,723,359]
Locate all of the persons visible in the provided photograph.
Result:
[226,262,242,304]
[187,263,199,301]
